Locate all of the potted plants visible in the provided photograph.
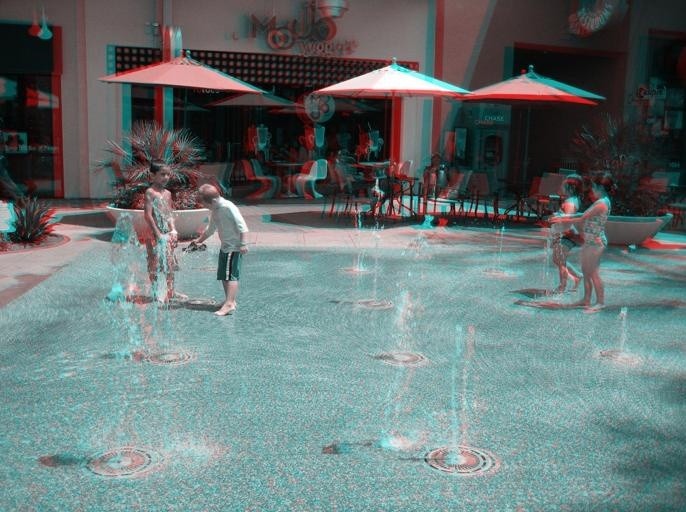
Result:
[571,112,674,245]
[91,119,215,241]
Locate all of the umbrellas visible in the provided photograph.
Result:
[98,49,264,144]
[453,63,607,195]
[313,58,475,164]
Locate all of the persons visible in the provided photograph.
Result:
[549,173,583,293]
[191,184,249,316]
[548,176,619,311]
[145,159,186,300]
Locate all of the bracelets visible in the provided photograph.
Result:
[240,242,247,246]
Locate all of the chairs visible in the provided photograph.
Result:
[640,171,686,229]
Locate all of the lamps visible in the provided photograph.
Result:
[310,0,350,31]
[37,4,53,40]
